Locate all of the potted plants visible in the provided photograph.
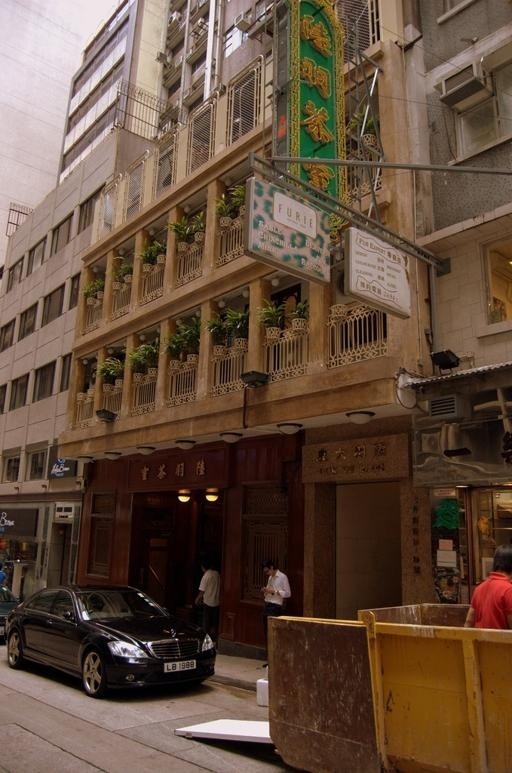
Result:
[99,286,311,395]
[76,181,245,307]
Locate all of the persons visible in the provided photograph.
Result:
[259,559,292,667]
[193,559,221,633]
[0,562,6,585]
[464,543,512,630]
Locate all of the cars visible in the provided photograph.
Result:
[4,580,217,700]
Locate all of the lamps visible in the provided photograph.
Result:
[345,410,376,424]
[76,453,93,462]
[218,431,243,444]
[175,439,195,450]
[136,445,155,456]
[276,422,302,435]
[103,450,122,461]
